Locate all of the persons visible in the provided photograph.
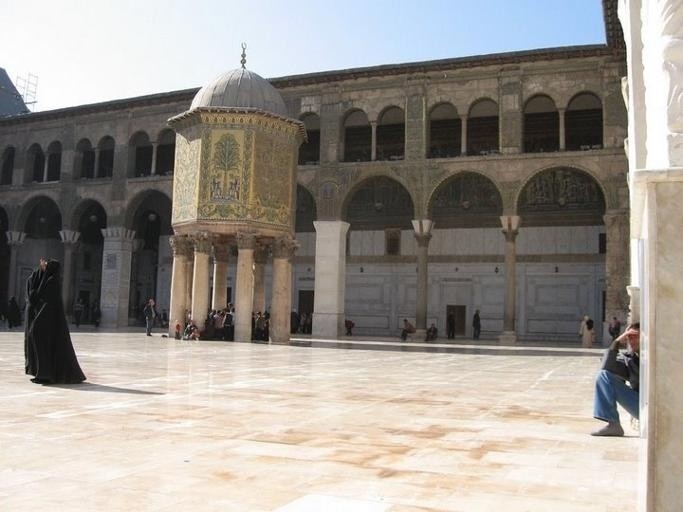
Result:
[345,320,354,336]
[589,321,641,436]
[129,299,167,337]
[291,308,313,335]
[610,317,621,353]
[448,311,456,338]
[580,315,593,347]
[28,256,87,383]
[473,309,480,339]
[6,296,21,329]
[425,324,437,341]
[24,256,49,374]
[72,296,99,328]
[176,302,269,341]
[400,319,416,340]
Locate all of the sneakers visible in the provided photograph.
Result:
[590,425,624,437]
